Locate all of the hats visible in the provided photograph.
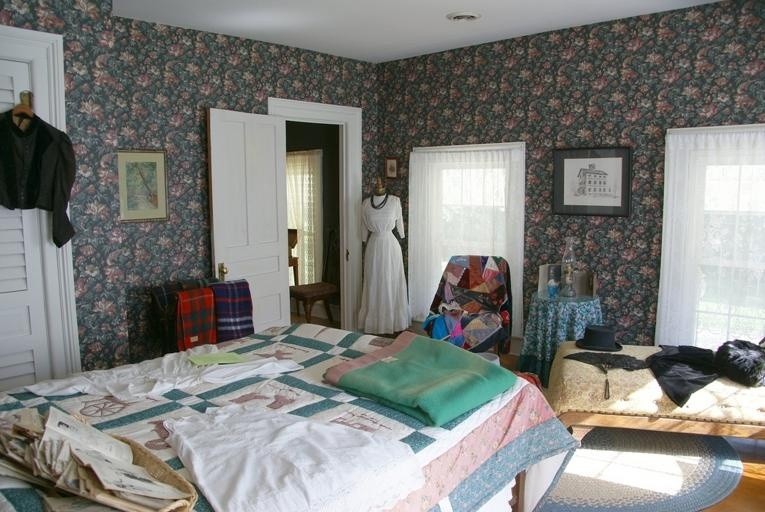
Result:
[575,325,624,351]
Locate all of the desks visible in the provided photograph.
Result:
[521,291,604,390]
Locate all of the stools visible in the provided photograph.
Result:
[289,281,338,326]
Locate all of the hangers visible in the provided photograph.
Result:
[11,89,34,120]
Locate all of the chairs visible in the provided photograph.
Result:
[424,255,512,355]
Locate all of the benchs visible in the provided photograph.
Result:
[548,342,765,444]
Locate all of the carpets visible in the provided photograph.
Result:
[523,425,744,512]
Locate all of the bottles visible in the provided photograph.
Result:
[560,237,575,298]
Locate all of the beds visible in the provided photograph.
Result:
[0,316,582,512]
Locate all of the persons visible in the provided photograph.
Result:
[358,178,414,337]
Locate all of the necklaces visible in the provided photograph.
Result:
[370,192,389,209]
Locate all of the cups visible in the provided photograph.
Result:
[548,284,558,297]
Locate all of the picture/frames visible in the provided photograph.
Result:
[552,147,633,218]
[117,149,170,222]
